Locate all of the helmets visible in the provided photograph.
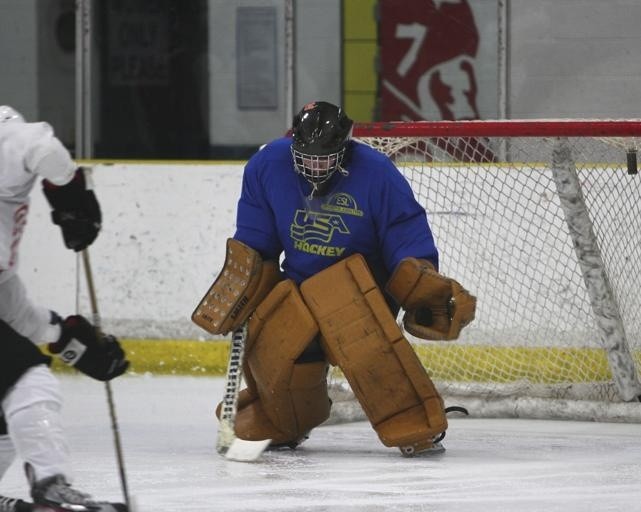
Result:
[289,101,353,184]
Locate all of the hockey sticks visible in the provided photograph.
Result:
[217,317,272,463]
[82,246,136,510]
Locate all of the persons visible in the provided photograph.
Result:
[217,127,295,422]
[232,101,448,453]
[0,104,128,511]
[0,266,132,510]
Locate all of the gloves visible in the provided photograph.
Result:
[48,314,128,381]
[41,167,100,251]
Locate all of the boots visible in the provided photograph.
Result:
[23,461,127,512]
